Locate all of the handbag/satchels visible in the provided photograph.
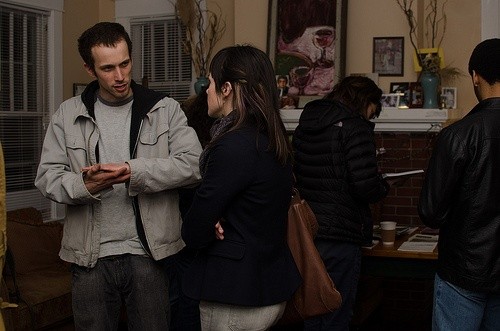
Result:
[321,251,366,288]
[285,187,343,319]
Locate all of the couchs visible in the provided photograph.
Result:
[0,207,72,331]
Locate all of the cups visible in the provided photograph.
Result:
[380,221,397,244]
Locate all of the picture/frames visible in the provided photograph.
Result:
[372,36,403,76]
[441,87,457,109]
[409,81,423,108]
[389,82,411,106]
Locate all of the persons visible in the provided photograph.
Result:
[291,75,398,331]
[417,37,500,331]
[277,76,289,97]
[174,44,302,331]
[35,21,204,331]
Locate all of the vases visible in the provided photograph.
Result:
[420,69,442,108]
[195,77,208,92]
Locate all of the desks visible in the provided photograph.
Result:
[362,223,437,259]
